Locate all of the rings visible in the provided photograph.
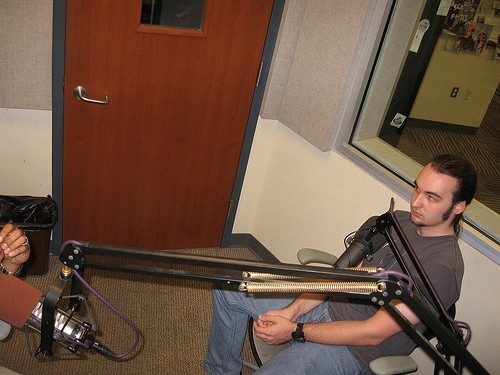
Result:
[22,236,28,246]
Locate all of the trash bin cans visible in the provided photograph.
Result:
[0,194,59,275]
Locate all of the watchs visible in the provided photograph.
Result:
[292,323,305,343]
[0,261,23,277]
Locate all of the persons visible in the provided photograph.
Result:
[204,155,478,375]
[0,223,30,342]
[456,25,475,51]
[474,26,487,53]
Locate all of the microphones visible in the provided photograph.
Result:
[333,214,390,270]
[0,268,93,353]
